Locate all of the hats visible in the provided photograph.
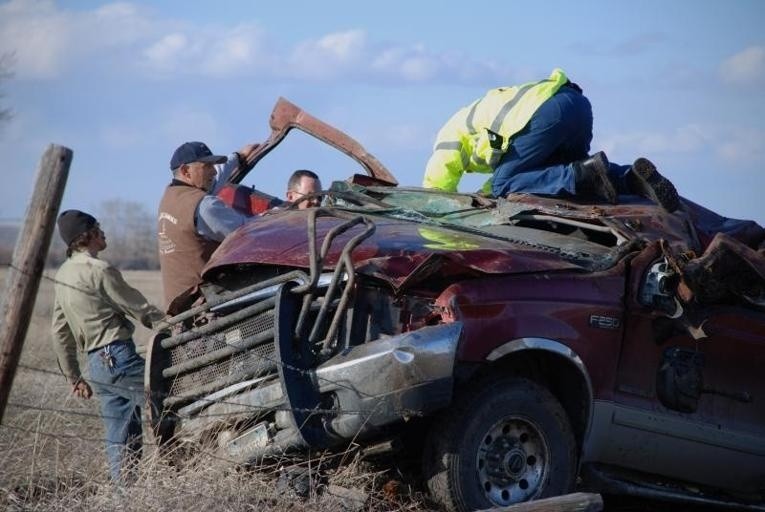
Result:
[170,141,228,170]
[57,209,96,247]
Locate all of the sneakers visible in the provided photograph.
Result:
[633,158,679,212]
[583,151,618,204]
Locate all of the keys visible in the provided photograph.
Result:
[100,352,118,374]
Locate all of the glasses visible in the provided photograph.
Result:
[291,191,323,201]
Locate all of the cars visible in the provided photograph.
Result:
[144,172,761,508]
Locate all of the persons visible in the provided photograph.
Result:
[286,170,325,211]
[422,68,679,215]
[158,141,249,313]
[48,209,165,486]
